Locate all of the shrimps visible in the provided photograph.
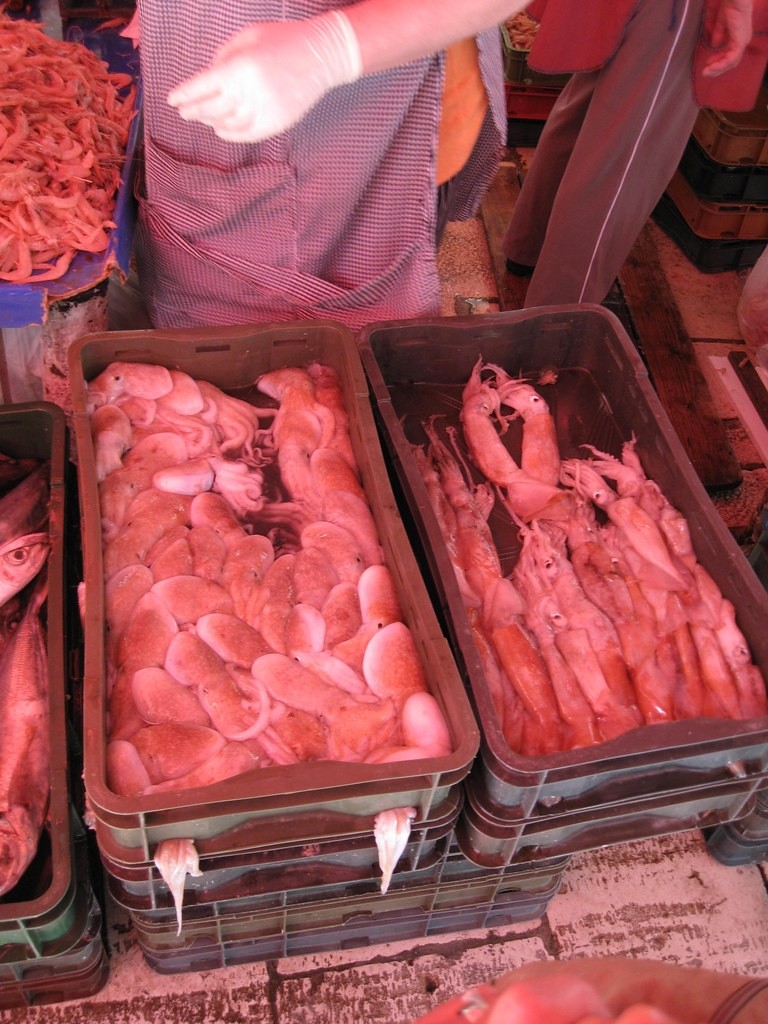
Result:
[431,366,759,757]
[0,4,139,288]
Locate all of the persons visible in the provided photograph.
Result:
[118,0,535,332]
[501,0,752,308]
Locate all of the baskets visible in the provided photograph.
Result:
[499,24,573,85]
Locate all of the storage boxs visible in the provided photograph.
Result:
[650,88,768,277]
[498,21,572,148]
[0,301,768,1007]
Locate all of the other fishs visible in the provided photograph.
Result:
[1,452,50,893]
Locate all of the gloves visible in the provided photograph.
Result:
[167,8,361,144]
